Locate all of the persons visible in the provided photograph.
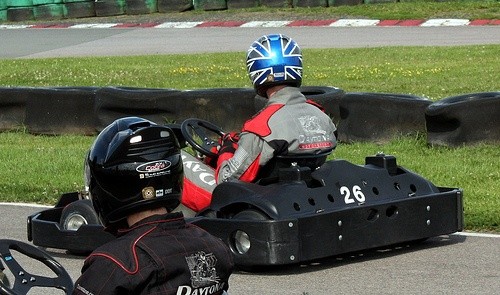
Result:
[71,116,233,295]
[179,33,338,214]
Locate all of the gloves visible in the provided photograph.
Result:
[218,132,240,154]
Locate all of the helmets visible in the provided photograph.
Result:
[246,34,303,100]
[84,117,184,228]
